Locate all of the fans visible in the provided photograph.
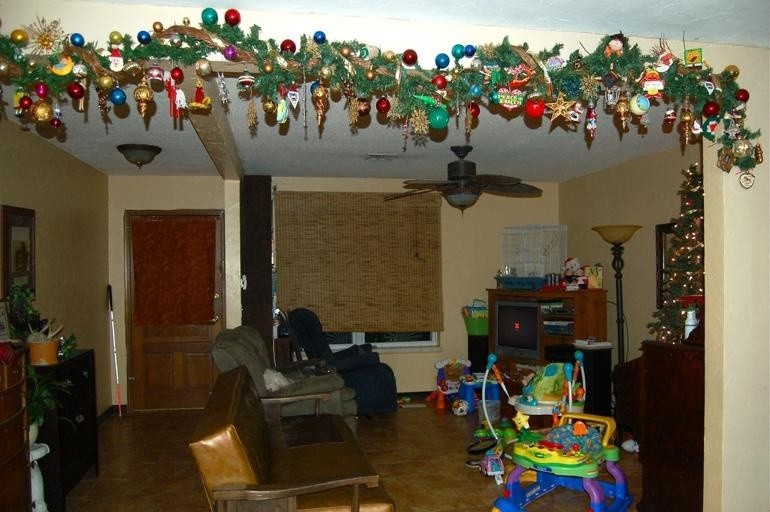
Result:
[379,144,544,199]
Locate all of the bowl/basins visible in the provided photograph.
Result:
[29,341,58,365]
[565,275,588,292]
[464,316,490,336]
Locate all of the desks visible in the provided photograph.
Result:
[30,442,52,512]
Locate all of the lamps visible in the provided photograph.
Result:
[442,190,480,215]
[118,144,161,169]
[591,223,643,363]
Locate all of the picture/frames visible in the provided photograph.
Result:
[0,204,36,303]
[654,222,672,309]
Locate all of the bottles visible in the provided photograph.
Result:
[545,273,561,287]
[685,310,699,339]
[497,264,512,277]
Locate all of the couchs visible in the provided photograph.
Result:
[188,363,396,512]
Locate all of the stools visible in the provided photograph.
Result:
[457,374,500,415]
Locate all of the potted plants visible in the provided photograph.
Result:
[29,363,72,446]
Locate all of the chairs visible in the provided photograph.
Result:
[278,309,397,421]
[212,324,361,435]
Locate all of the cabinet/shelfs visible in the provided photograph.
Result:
[467,335,488,374]
[547,347,611,418]
[1,339,35,512]
[541,289,608,359]
[29,349,99,512]
[635,338,703,512]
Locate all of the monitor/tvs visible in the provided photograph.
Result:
[495,301,539,360]
